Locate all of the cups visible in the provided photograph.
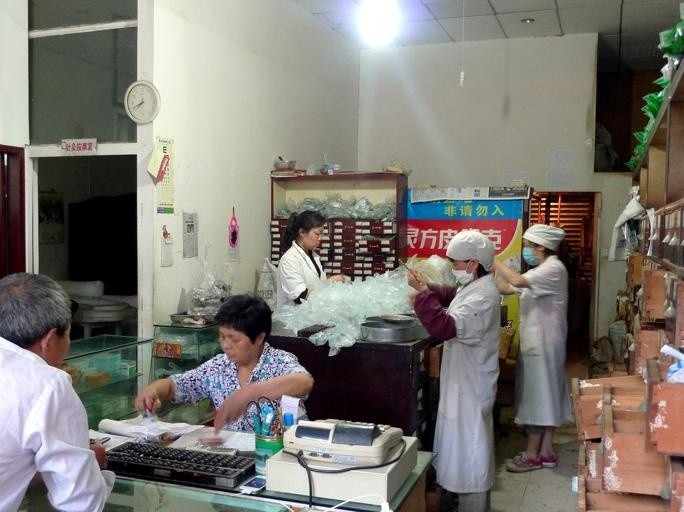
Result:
[255,432,283,479]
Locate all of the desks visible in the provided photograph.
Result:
[104,427,438,511]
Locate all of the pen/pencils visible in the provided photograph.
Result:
[253,404,286,437]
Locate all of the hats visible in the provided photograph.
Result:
[522,224,565,251]
[446,231,495,272]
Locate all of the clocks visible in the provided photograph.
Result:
[123,80,161,125]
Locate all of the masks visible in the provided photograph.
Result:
[452,261,475,285]
[523,247,542,266]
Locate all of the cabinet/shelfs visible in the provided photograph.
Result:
[271,170,410,280]
[569,56,684,512]
[27,334,153,511]
[271,322,446,453]
[152,316,221,424]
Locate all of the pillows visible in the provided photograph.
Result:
[53,278,105,298]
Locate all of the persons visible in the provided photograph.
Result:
[493,223,574,473]
[407,231,501,512]
[133,293,314,437]
[0,272,116,512]
[277,210,345,312]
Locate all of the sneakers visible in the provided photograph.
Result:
[506,453,556,473]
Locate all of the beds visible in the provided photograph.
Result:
[65,295,137,341]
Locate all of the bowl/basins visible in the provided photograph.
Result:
[274,161,296,171]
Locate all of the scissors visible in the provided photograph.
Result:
[244,396,275,432]
